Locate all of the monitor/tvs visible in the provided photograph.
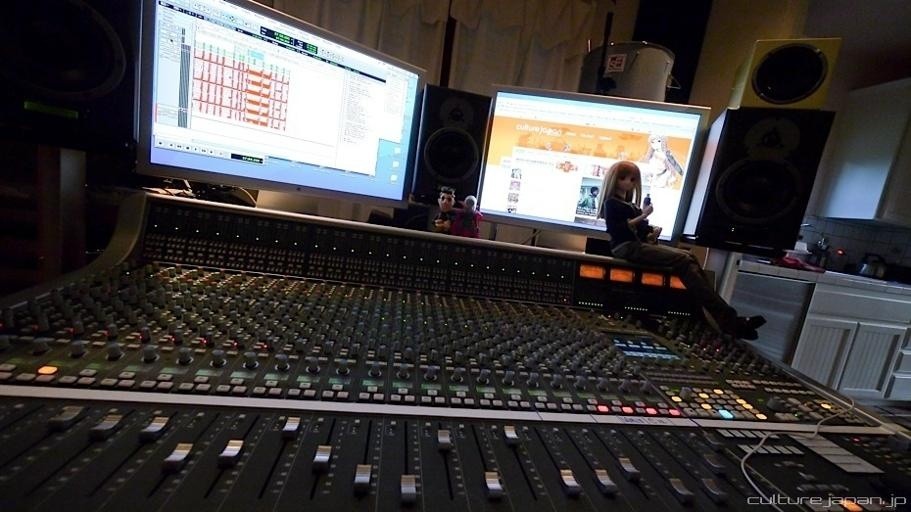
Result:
[475,84,712,247]
[136,0,427,211]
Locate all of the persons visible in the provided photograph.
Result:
[579,186,598,210]
[432,185,483,238]
[593,159,767,342]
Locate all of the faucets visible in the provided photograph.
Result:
[800,223,815,227]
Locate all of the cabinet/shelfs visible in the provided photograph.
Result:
[811,76,911,229]
[728,271,816,366]
[789,285,911,405]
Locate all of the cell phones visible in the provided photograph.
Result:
[758,258,775,265]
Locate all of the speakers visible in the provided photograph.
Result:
[409,84,494,213]
[683,108,836,258]
[727,38,841,111]
[0,0,142,156]
